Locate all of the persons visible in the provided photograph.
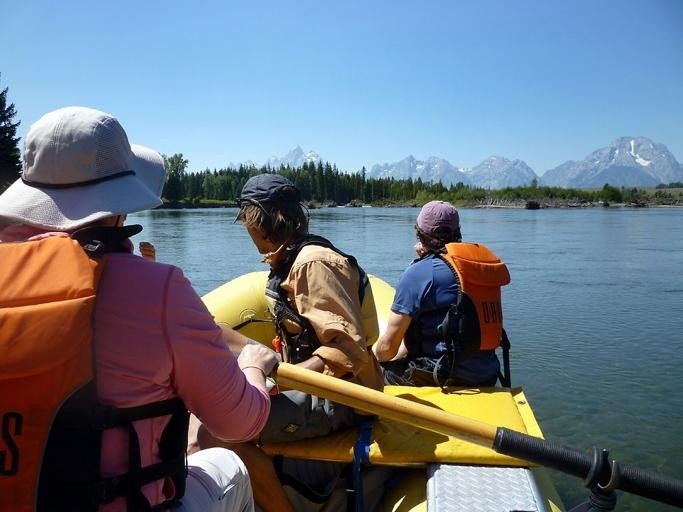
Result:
[0,106,278,512]
[198,175,382,512]
[377,200,510,387]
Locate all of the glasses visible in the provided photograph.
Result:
[237,198,270,216]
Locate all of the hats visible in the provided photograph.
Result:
[0,106,167,232]
[416,200,459,235]
[233,173,300,224]
